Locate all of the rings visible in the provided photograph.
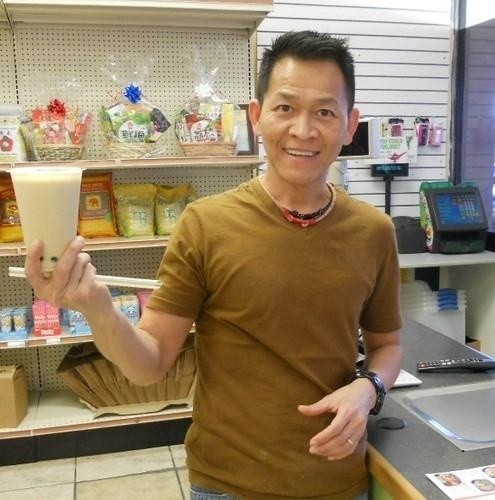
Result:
[347,439,354,445]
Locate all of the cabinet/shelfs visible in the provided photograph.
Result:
[0,0,274,439]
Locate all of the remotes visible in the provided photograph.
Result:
[416,356,495,374]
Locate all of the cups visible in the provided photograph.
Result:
[5,167,85,273]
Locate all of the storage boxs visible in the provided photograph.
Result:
[401,281,468,345]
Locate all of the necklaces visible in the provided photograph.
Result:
[263,181,337,228]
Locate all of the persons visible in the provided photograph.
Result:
[22,28,406,500]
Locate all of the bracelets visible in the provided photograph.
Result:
[348,369,386,417]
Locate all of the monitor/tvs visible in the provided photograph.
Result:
[427,185,489,233]
